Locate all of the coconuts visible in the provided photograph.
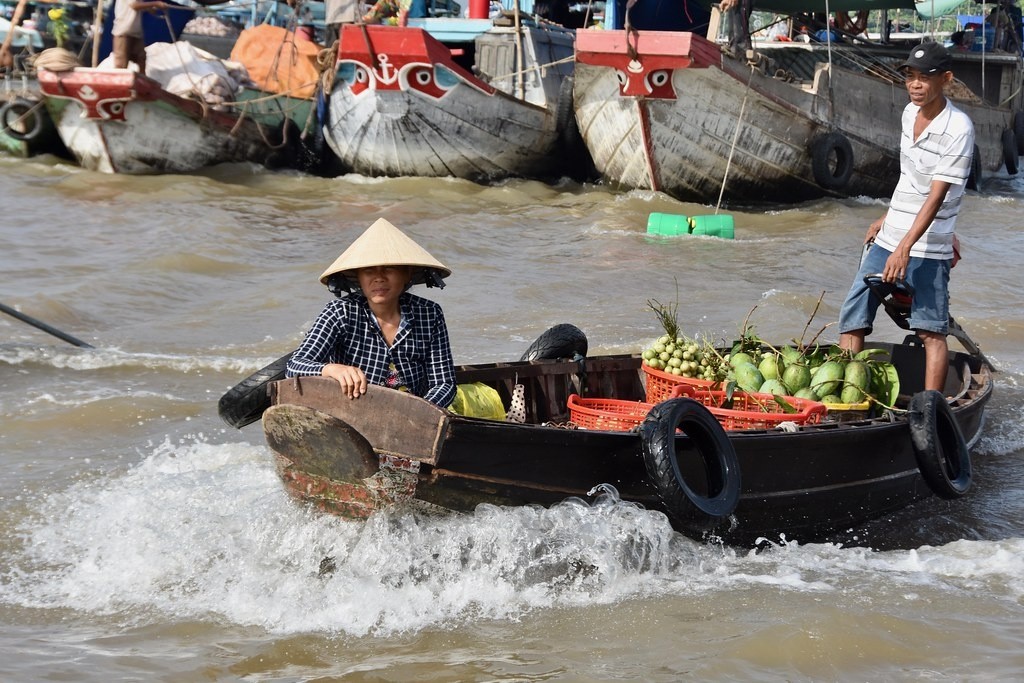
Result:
[730,352,869,404]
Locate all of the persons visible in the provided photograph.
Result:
[837,42,975,393]
[720,0,757,51]
[285,218,457,409]
[949,31,970,53]
[111,0,170,76]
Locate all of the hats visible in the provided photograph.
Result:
[897,42,952,76]
[318,218,451,290]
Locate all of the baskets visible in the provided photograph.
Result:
[567,393,656,432]
[642,353,726,402]
[671,384,827,431]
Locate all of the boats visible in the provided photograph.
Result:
[0,1,345,175]
[571,1,1024,213]
[320,1,575,183]
[217,229,997,546]
